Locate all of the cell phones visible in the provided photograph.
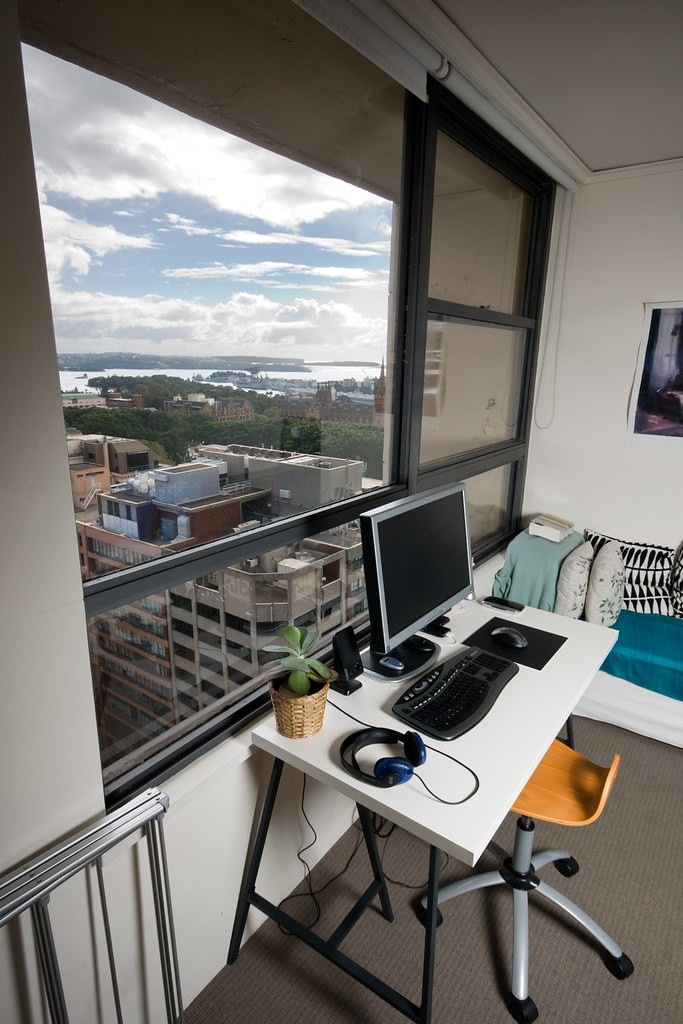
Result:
[483,596,525,612]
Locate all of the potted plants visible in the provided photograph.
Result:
[261,626,339,739]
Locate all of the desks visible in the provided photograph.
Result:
[228,596,619,1023]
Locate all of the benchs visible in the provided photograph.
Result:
[568,608,683,748]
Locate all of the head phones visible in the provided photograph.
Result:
[339,727,427,788]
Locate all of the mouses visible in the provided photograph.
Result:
[491,627,528,648]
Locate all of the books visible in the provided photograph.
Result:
[528,513,574,543]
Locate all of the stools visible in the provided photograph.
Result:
[412,735,636,1024]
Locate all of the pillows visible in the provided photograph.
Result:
[671,545,683,619]
[553,541,595,620]
[583,529,675,617]
[585,540,625,628]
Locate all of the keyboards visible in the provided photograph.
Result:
[392,646,519,741]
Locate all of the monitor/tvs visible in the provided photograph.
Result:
[359,481,475,684]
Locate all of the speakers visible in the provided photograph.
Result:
[328,625,364,696]
